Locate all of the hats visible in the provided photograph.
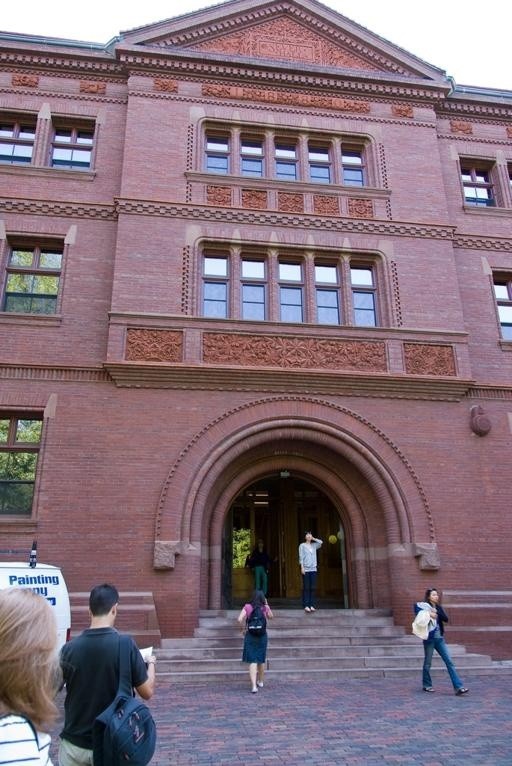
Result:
[255,538,265,544]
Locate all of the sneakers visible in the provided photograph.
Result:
[305,605,316,613]
[251,678,264,693]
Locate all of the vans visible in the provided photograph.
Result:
[0,537,72,650]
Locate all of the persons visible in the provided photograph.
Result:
[238,590,273,693]
[412,587,469,696]
[0,586,64,766]
[250,539,270,598]
[59,582,156,766]
[298,529,324,612]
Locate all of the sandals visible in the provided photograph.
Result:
[422,686,436,693]
[455,687,470,696]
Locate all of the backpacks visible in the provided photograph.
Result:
[245,602,268,636]
[91,633,157,766]
